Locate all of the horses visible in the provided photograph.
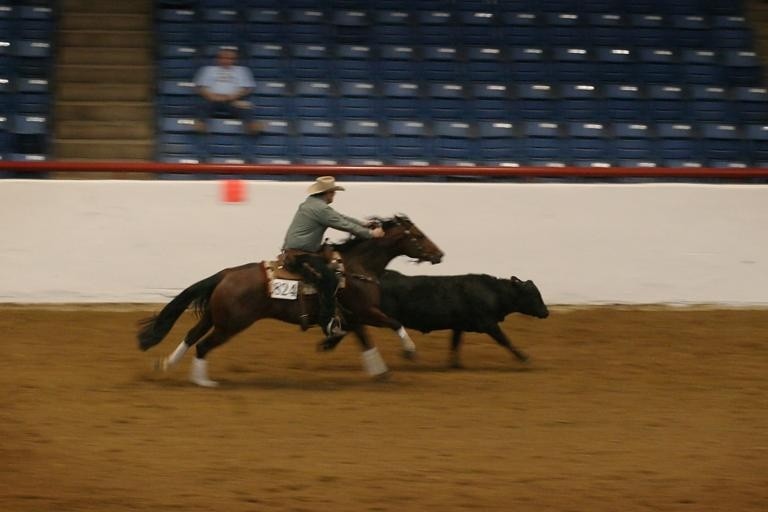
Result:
[134,213,444,389]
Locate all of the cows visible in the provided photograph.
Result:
[315,264,551,371]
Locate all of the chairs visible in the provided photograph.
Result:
[0,0,57,161]
[156,0,768,167]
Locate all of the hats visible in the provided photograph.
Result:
[306,176,345,196]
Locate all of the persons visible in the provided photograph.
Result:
[191,37,268,141]
[283,168,388,351]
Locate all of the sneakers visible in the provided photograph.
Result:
[332,326,347,336]
